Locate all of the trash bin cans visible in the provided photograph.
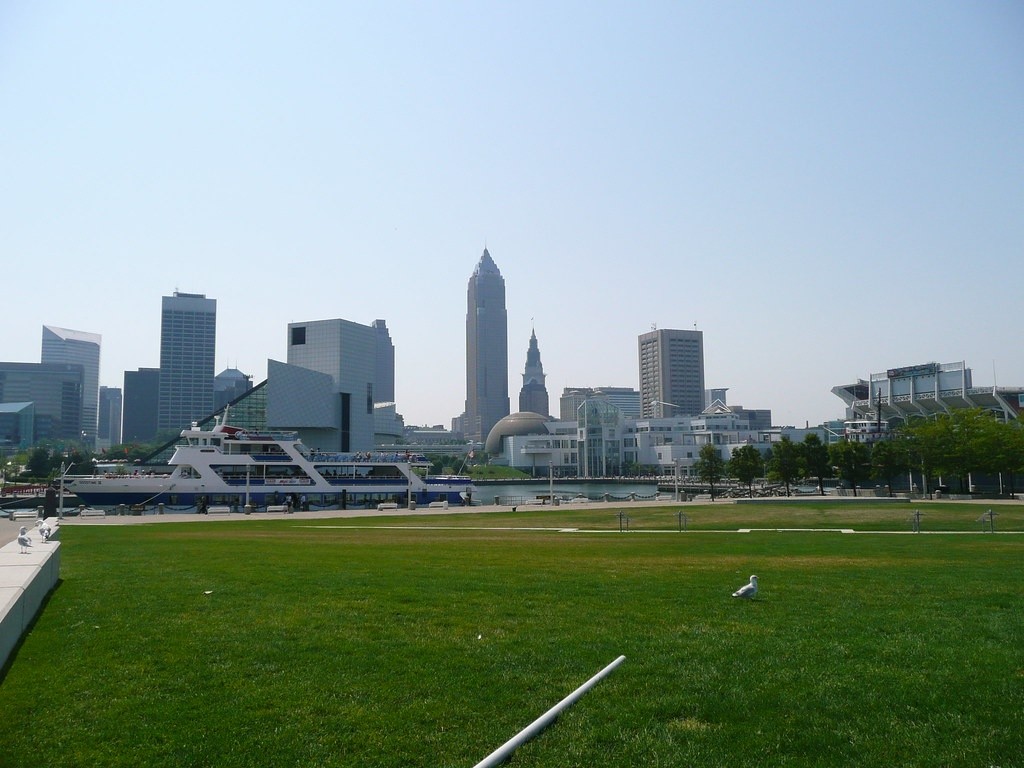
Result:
[554,499,559,506]
[494,496,499,505]
[411,501,416,510]
[244,505,251,515]
[935,490,941,499]
[681,490,685,501]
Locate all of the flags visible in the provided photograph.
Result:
[469,447,474,457]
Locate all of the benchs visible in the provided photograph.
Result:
[656,495,672,503]
[465,499,482,507]
[571,498,589,506]
[81,509,105,519]
[429,501,449,510]
[12,510,39,521]
[207,506,230,517]
[267,505,288,513]
[378,503,398,511]
[527,499,544,506]
[695,494,712,501]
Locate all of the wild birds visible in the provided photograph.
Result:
[34,520,51,543]
[17,526,32,554]
[732,575,759,602]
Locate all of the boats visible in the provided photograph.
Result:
[55,403,482,516]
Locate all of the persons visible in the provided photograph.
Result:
[466,486,471,505]
[355,449,410,462]
[134,469,138,475]
[218,469,223,477]
[104,470,107,475]
[309,447,322,462]
[182,470,188,475]
[324,468,361,476]
[286,494,305,514]
[197,496,207,515]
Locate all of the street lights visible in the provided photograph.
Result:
[548,460,555,506]
[245,463,252,514]
[407,460,412,511]
[59,461,65,520]
[671,456,679,504]
